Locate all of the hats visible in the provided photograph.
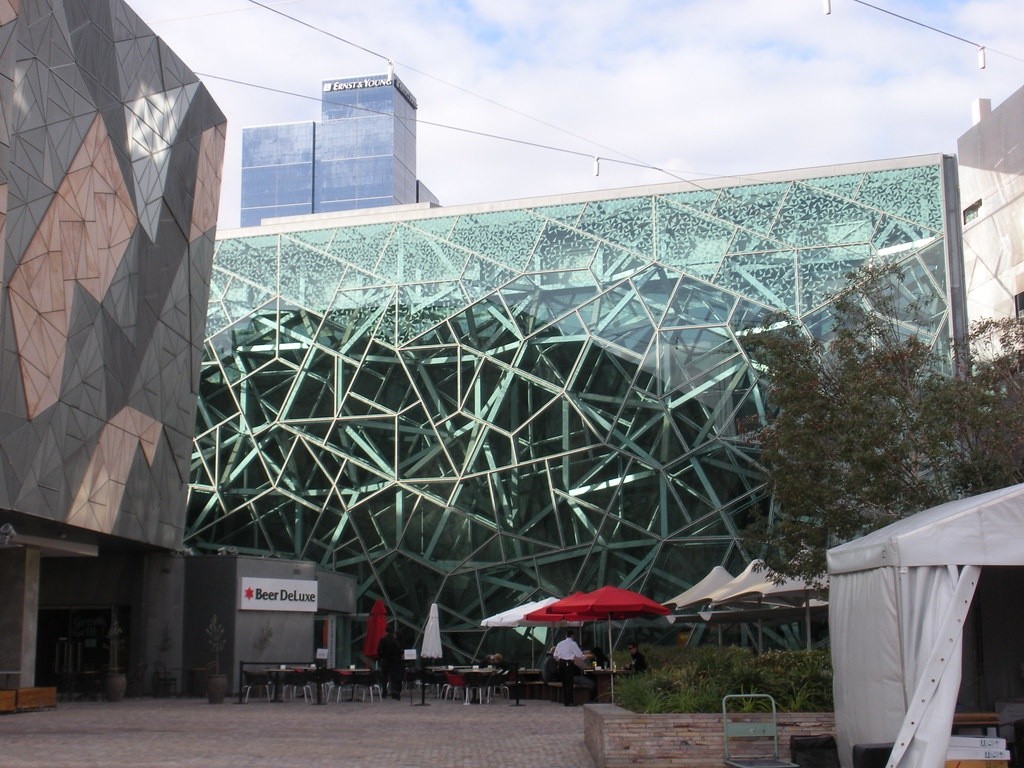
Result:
[548,646,555,654]
[564,630,576,635]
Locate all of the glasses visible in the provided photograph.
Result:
[629,647,634,650]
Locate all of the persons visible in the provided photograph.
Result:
[545,630,611,707]
[623,641,646,674]
[470,653,509,705]
[377,626,404,701]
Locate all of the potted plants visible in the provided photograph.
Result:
[104,619,127,702]
[152,625,178,698]
[205,615,227,704]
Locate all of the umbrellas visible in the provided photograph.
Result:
[363,599,389,671]
[420,603,442,666]
[480,584,672,670]
[662,558,828,654]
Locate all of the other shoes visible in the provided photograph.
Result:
[565,701,577,706]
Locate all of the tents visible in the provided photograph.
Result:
[825,484,1024,768]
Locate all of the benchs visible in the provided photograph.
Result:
[522,680,586,703]
[0,690,16,714]
[5,687,56,711]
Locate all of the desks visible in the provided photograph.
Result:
[515,668,628,705]
[952,711,999,736]
[0,671,22,687]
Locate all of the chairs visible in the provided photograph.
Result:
[722,694,800,768]
[53,636,102,701]
[852,742,896,768]
[238,665,515,707]
[788,734,842,768]
